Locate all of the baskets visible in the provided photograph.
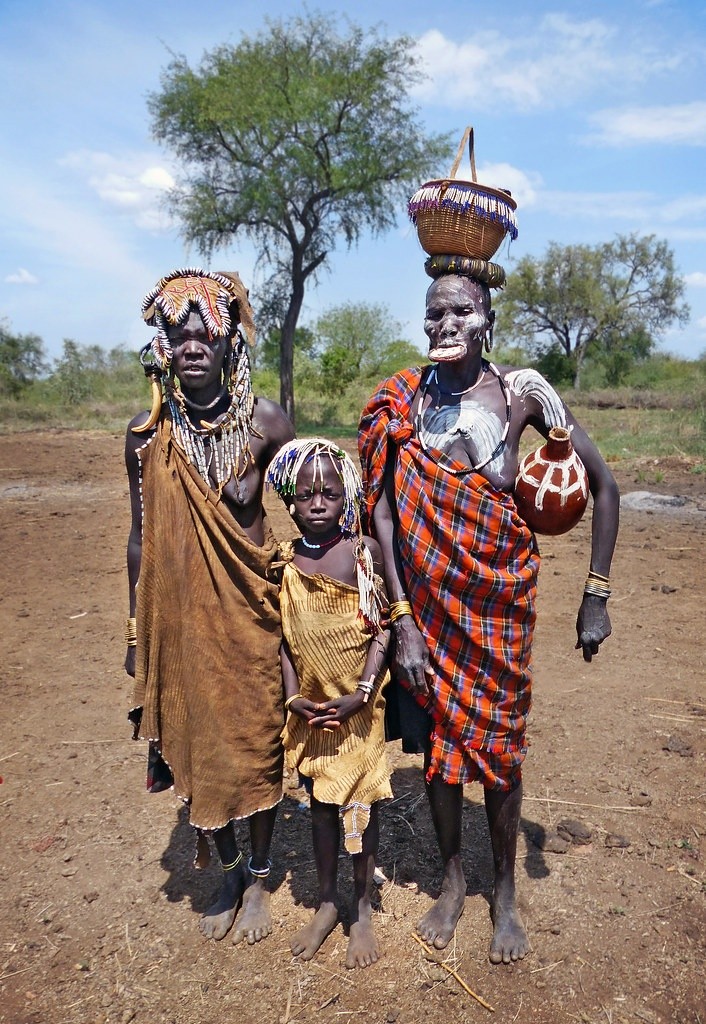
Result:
[406,127,519,261]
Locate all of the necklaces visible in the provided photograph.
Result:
[433,366,487,410]
[302,526,345,549]
[417,356,511,473]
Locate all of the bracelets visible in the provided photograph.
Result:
[584,571,611,600]
[284,694,303,710]
[388,601,414,624]
[356,680,374,696]
[124,617,137,647]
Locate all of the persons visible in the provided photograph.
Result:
[123,268,298,946]
[264,438,393,970]
[358,256,621,965]
